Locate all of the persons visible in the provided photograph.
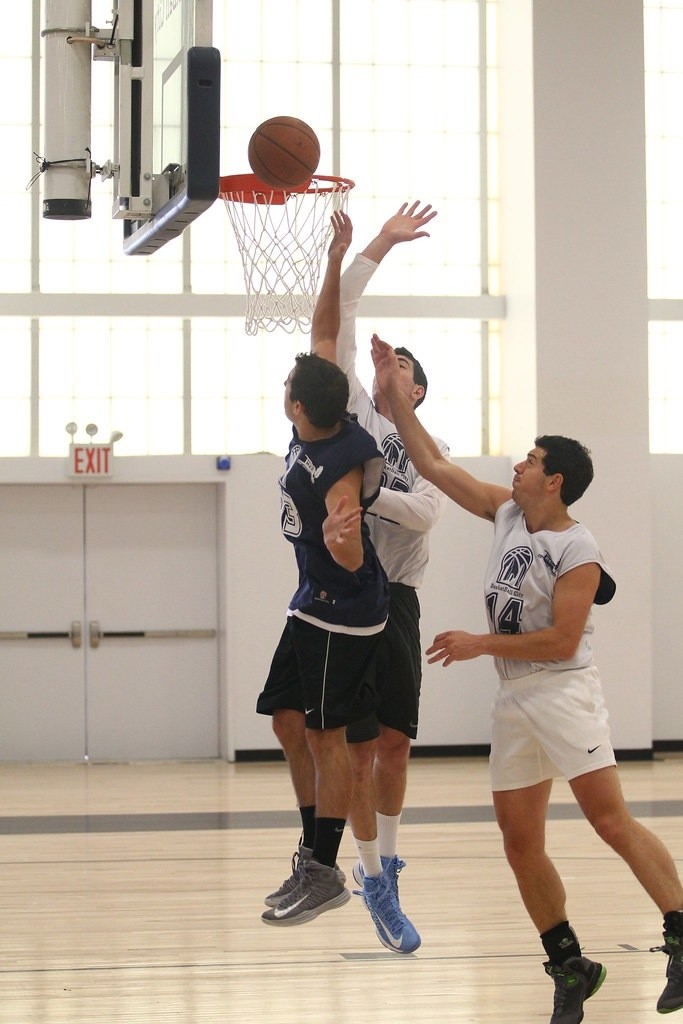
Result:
[333,195,458,957]
[368,329,683,1023]
[252,205,394,930]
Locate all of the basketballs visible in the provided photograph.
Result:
[246,117,320,191]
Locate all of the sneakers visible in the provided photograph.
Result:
[352,854,405,909]
[361,872,421,955]
[264,846,347,907]
[261,861,351,927]
[544,955,607,1024]
[657,930,683,1013]
